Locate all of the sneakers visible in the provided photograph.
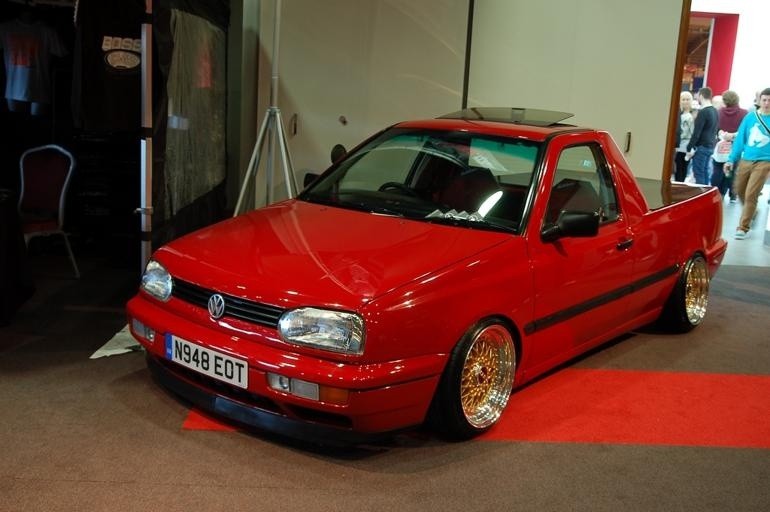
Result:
[735,230,750,239]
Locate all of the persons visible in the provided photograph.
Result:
[723,88,770,240]
[670,88,760,204]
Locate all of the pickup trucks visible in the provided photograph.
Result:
[125,105,728,442]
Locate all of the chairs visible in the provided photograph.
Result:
[16,144,84,279]
[436,163,601,228]
